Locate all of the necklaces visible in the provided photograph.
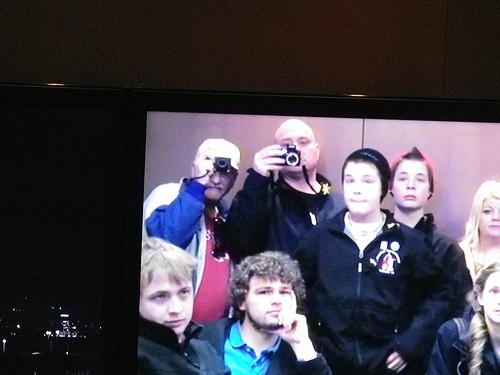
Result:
[346,210,384,236]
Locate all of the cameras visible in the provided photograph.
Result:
[276,145,301,167]
[213,157,231,173]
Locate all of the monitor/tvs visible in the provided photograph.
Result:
[0,82,500,375]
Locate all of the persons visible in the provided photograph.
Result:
[292,147,455,375]
[136,115,499,375]
